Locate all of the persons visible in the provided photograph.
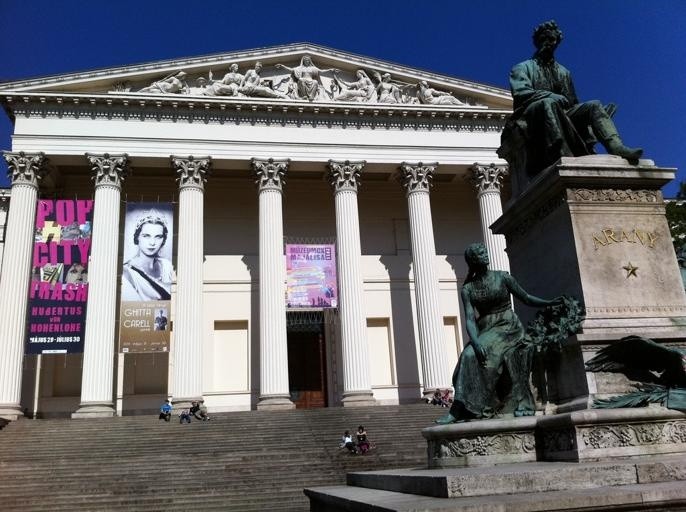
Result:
[441,389,452,408]
[367,68,405,102]
[331,68,374,102]
[63,263,89,284]
[119,207,174,303]
[431,388,441,406]
[137,69,187,95]
[178,408,191,424]
[190,401,210,421]
[159,399,172,422]
[504,17,644,166]
[406,77,465,105]
[206,61,244,97]
[274,52,344,100]
[356,425,370,454]
[341,429,359,456]
[194,400,208,421]
[153,310,168,331]
[238,60,289,101]
[432,241,566,423]
